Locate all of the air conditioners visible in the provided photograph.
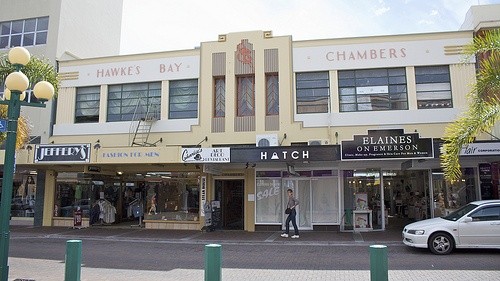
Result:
[307,138,330,146]
[255,132,279,147]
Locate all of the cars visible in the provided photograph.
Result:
[401,199,500,255]
[11,197,36,217]
[61,199,90,218]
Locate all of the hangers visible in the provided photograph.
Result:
[91,198,112,207]
[129,199,141,207]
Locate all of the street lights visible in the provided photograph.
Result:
[0,46,55,281]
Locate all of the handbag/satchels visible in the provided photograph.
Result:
[285,208,292,214]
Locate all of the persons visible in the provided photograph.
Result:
[281,188,300,238]
[24,176,35,198]
[147,192,159,216]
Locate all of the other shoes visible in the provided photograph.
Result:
[290,235,299,238]
[281,234,289,237]
